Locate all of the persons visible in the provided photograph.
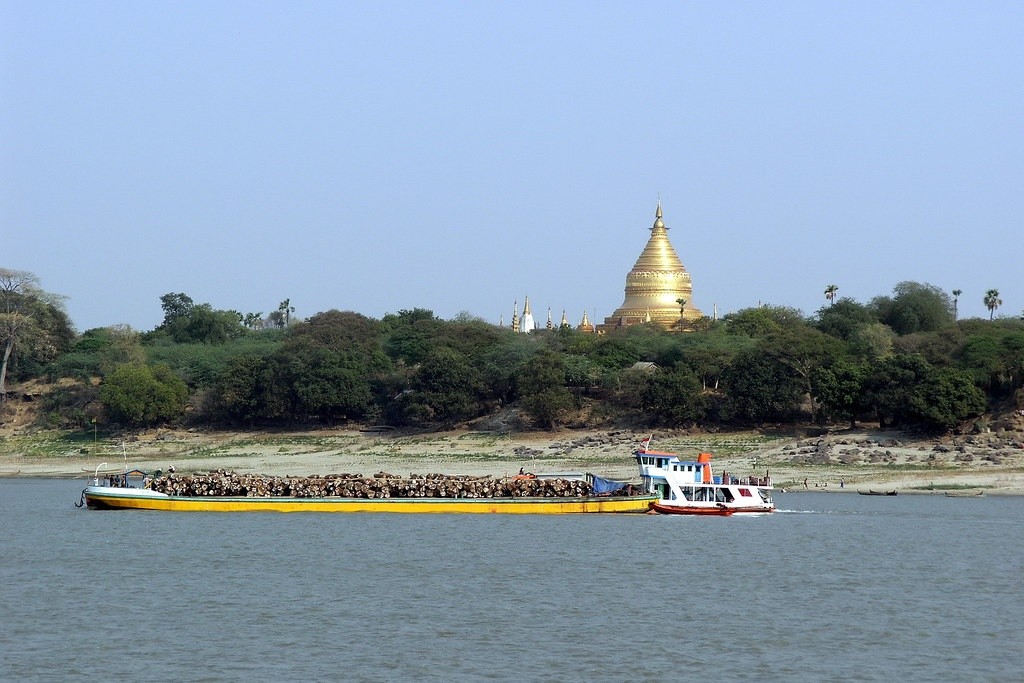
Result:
[782,487,786,492]
[155,469,162,477]
[804,478,808,489]
[686,491,702,500]
[104,474,125,488]
[520,467,524,474]
[841,478,844,488]
[143,476,152,489]
[168,465,175,473]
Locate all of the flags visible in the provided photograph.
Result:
[641,437,649,447]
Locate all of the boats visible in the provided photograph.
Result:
[658,482,777,513]
[648,502,738,516]
[585,447,775,495]
[81,466,663,514]
